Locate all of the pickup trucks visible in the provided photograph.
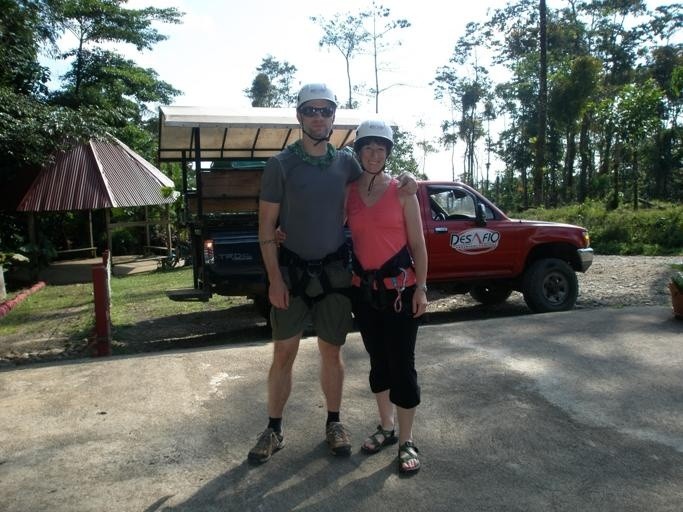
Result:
[158,175,596,327]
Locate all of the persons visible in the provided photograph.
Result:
[248,82,419,464]
[272,118,429,472]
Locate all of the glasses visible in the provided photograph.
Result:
[301,106,333,117]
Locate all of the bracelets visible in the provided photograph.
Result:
[416,284,428,292]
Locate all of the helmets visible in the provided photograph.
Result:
[354,121,394,154]
[297,83,337,111]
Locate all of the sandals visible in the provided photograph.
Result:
[399,442,420,472]
[361,426,399,453]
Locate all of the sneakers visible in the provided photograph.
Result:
[325,422,352,457]
[248,428,284,462]
